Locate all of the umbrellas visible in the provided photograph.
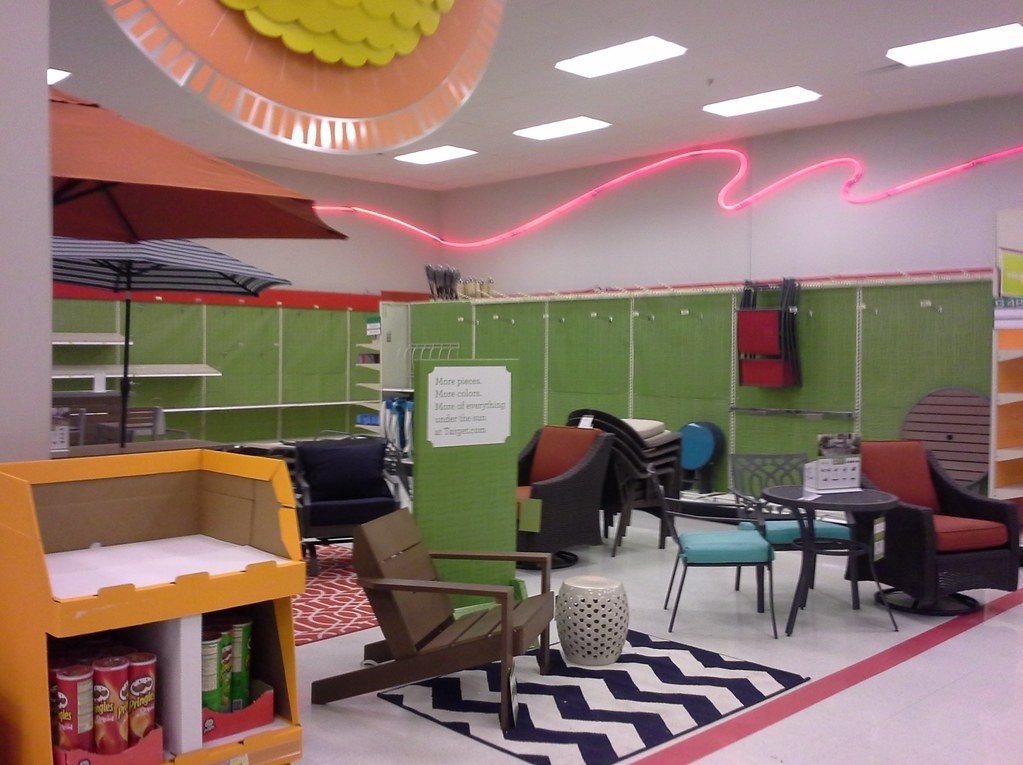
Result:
[47,83,350,246]
[52,235,292,450]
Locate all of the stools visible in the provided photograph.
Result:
[679,421,725,494]
[555,575,630,666]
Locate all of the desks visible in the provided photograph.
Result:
[762,486,899,637]
[903,389,990,489]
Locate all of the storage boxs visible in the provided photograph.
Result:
[51,721,163,765]
[200,680,274,744]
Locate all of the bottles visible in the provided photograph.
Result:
[383,397,415,459]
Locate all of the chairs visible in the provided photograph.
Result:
[312,507,554,732]
[728,453,859,613]
[648,462,778,640]
[517,426,615,571]
[737,278,802,389]
[51,390,189,446]
[566,409,682,558]
[296,437,401,576]
[844,442,1019,616]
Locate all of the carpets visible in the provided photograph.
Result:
[291,545,379,646]
[377,629,811,765]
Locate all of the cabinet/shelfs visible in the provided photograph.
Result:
[354,301,411,438]
[991,319,1023,566]
[382,389,414,499]
[51,333,381,449]
[0,448,307,765]
